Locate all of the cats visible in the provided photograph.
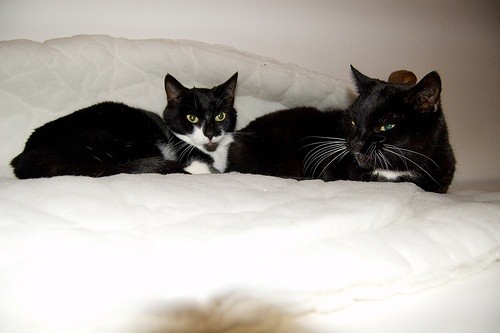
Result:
[224,63,462,194]
[8,70,257,181]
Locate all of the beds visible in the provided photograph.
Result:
[0,34,500,333]
